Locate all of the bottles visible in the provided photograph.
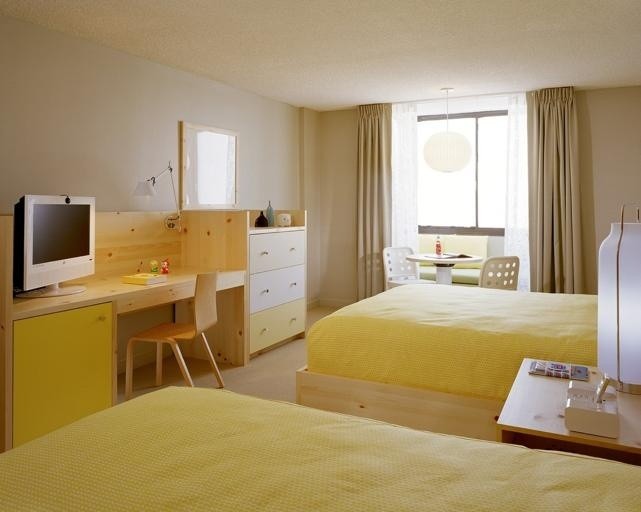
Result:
[435,234,442,255]
[254,199,275,228]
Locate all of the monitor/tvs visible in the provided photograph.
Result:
[13,195,96,298]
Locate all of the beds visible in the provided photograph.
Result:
[297,283,599,443]
[0,387,641,512]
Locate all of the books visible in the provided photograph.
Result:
[122,272,167,285]
[528,361,589,382]
[424,252,472,260]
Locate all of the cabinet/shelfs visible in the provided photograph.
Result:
[174,206,306,366]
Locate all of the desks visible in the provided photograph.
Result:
[0,266,245,452]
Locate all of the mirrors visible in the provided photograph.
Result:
[179,121,240,209]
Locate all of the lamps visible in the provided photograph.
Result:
[598,204,641,395]
[422,87,472,175]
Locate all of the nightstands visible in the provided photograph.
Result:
[497,358,641,468]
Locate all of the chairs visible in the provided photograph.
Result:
[125,271,225,398]
[382,246,519,290]
